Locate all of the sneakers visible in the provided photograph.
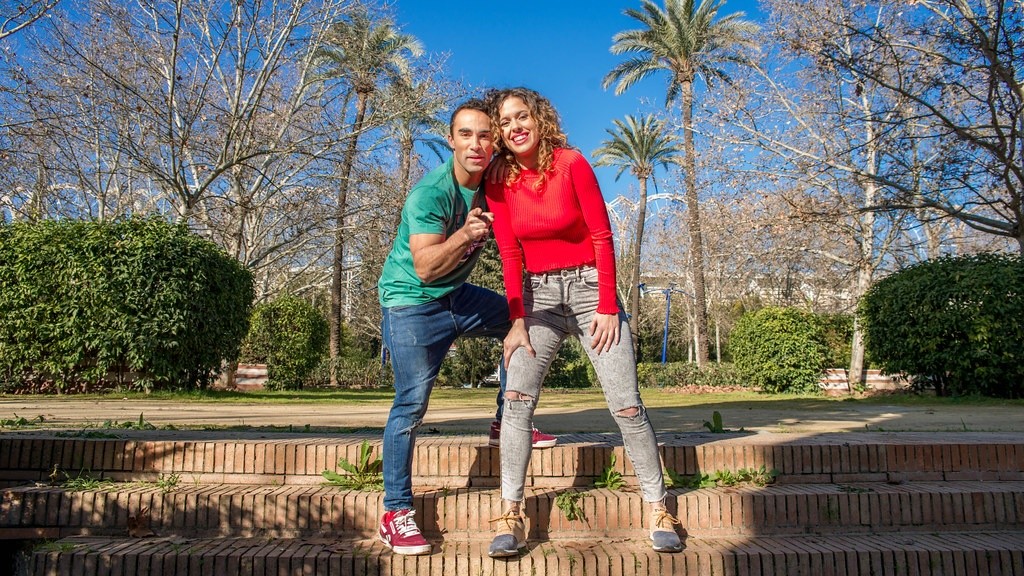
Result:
[488,420,557,448]
[378,509,431,555]
[487,505,526,557]
[648,506,682,551]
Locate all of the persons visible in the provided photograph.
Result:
[377,100,557,554]
[484,87,681,557]
